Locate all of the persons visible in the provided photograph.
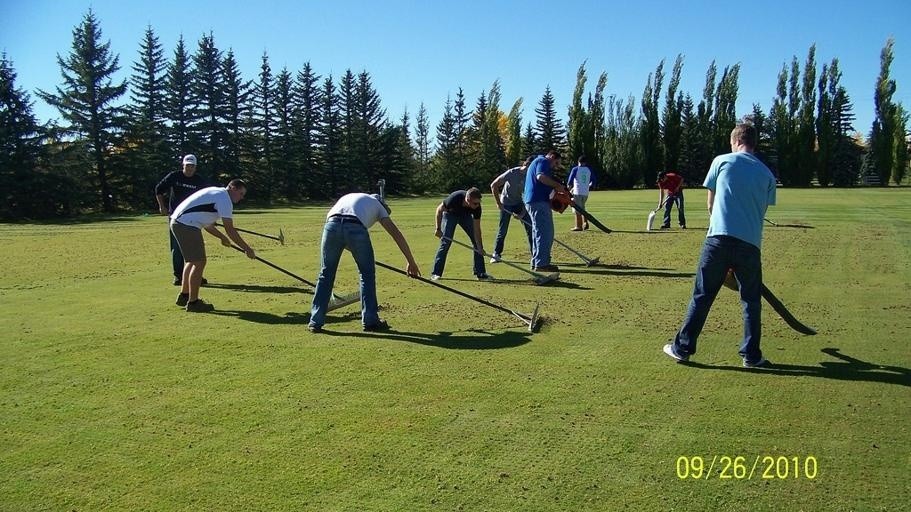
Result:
[307,192,421,332]
[656,171,686,229]
[566,155,596,230]
[169,179,255,311]
[489,155,535,263]
[523,151,567,271]
[663,125,776,367]
[430,187,493,280]
[154,154,207,285]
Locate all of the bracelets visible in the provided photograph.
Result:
[437,228,441,230]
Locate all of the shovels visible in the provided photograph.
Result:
[647,195,671,231]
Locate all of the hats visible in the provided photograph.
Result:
[657,171,666,183]
[183,154,198,166]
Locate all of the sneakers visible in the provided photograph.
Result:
[583,222,590,230]
[681,225,687,229]
[531,265,560,272]
[663,343,691,362]
[176,292,190,307]
[308,324,322,333]
[432,274,442,281]
[476,274,497,281]
[363,320,390,332]
[571,227,584,231]
[743,358,766,367]
[202,277,208,284]
[661,225,671,229]
[490,253,502,263]
[173,276,182,286]
[186,300,215,313]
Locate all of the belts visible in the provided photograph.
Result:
[328,218,363,223]
